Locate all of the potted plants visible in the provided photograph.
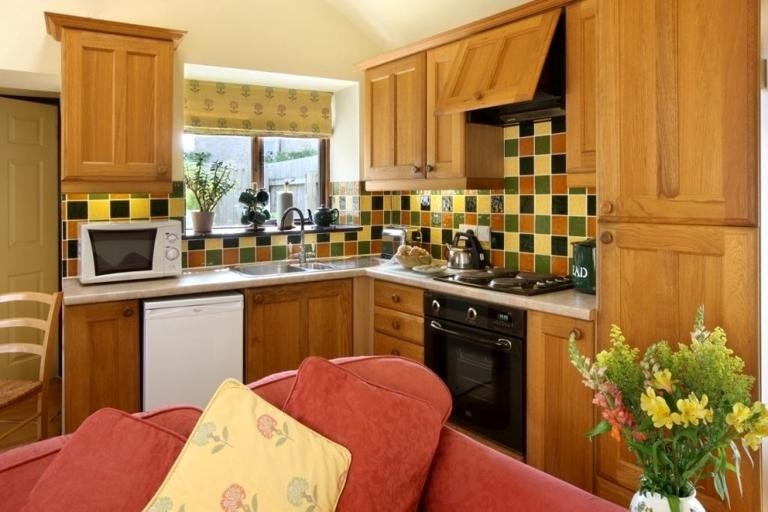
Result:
[184,152,236,232]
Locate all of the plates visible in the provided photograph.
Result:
[412,264,447,275]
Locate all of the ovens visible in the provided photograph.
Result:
[424,292,526,457]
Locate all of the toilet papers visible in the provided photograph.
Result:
[278,193,293,226]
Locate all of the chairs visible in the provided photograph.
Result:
[0,292,63,440]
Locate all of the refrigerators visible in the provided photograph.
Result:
[143,294,246,411]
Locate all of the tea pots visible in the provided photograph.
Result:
[308,205,340,227]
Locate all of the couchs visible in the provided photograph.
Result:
[0,355,631,512]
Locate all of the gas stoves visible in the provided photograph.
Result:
[433,267,576,297]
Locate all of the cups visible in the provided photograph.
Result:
[239,188,271,225]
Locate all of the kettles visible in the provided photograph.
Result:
[446,232,486,269]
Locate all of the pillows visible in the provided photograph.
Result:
[143,378,351,511]
[24,408,186,511]
[282,357,441,512]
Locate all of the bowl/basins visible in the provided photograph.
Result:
[397,254,434,269]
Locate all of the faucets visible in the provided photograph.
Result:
[280,207,305,248]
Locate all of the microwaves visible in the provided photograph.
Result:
[78,219,183,283]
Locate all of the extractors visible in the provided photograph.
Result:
[467,12,567,128]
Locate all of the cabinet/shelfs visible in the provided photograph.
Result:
[373,279,427,365]
[593,0,762,512]
[566,0,596,174]
[362,40,504,192]
[244,279,353,384]
[63,299,141,435]
[42,12,188,194]
[527,310,593,495]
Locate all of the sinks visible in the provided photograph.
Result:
[294,263,333,270]
[233,264,305,279]
